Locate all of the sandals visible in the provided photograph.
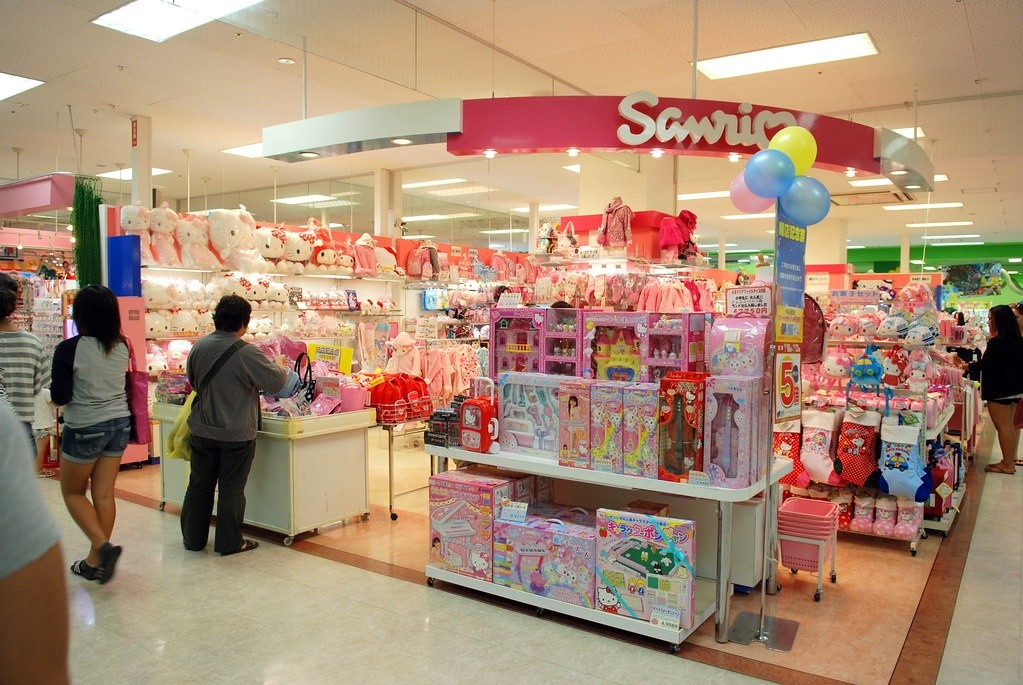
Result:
[36,468,55,478]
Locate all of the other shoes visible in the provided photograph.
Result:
[771,482,924,542]
[1014,458,1023,465]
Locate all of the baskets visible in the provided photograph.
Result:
[778,496,841,571]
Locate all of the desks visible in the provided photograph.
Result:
[150,402,377,548]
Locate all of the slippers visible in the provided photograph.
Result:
[984,463,1017,474]
[71,559,97,580]
[97,546,121,584]
[222,539,258,555]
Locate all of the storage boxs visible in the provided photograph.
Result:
[427,306,771,627]
[306,342,355,378]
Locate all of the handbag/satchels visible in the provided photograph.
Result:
[121,334,152,446]
[293,352,316,403]
[557,221,580,253]
[166,392,196,462]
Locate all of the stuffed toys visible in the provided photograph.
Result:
[146,339,193,404]
[122,201,269,274]
[240,314,275,341]
[558,235,575,256]
[140,271,291,310]
[360,298,396,315]
[302,287,350,310]
[800,311,936,395]
[144,308,216,336]
[384,332,422,378]
[279,310,355,337]
[256,224,355,275]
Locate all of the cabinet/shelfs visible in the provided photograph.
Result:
[108,204,407,467]
[804,384,929,557]
[372,396,448,521]
[802,300,969,540]
[394,238,540,351]
[537,210,756,315]
[420,441,796,651]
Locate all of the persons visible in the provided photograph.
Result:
[598,196,635,249]
[961,302,1023,474]
[0,273,74,684]
[51,285,130,585]
[429,537,457,571]
[563,395,586,459]
[180,294,287,555]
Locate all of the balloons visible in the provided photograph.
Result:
[731,125,830,225]
